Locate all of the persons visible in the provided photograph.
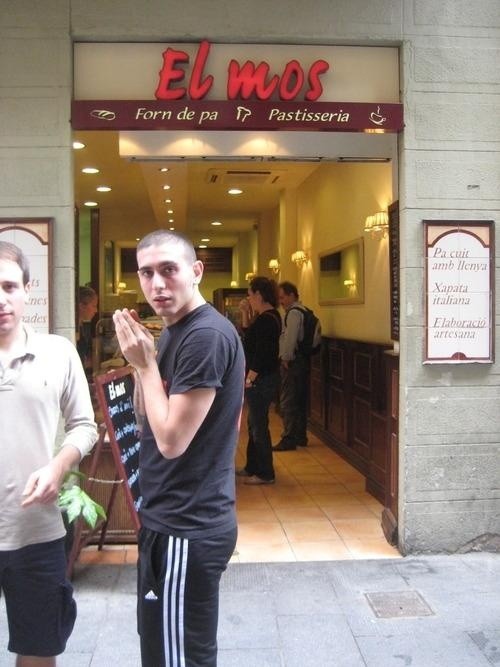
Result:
[234,275,282,485]
[112,226,246,667]
[272,280,310,452]
[0,236,102,667]
[77,284,98,370]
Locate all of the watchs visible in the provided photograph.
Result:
[245,378,254,385]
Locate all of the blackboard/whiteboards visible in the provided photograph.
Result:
[93,366,142,532]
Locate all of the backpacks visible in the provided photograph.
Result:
[284,306,322,356]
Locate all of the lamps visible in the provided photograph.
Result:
[363,210,389,240]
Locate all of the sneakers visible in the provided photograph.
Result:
[295,435,308,447]
[243,474,275,484]
[236,467,251,477]
[273,437,297,451]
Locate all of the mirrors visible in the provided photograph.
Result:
[317,236,366,305]
